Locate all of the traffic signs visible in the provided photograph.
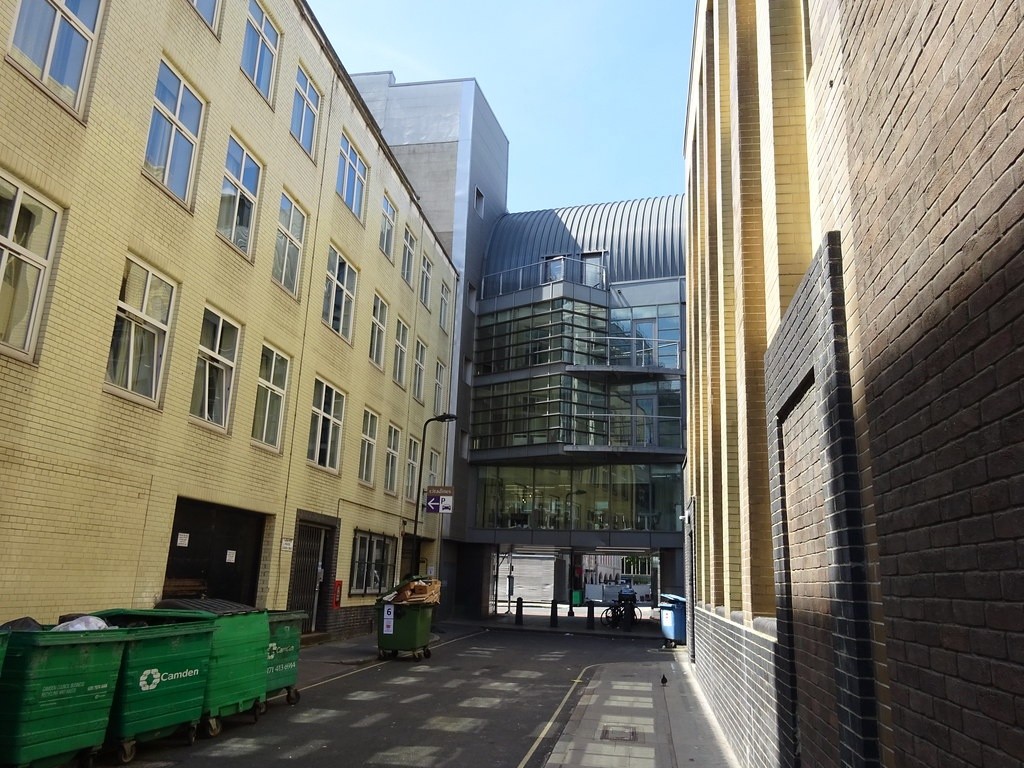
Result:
[425,486,454,513]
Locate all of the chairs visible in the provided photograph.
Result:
[652,512,662,530]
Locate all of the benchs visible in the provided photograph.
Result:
[162,578,208,598]
[611,439,648,446]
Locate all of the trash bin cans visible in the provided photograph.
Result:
[658,594,686,648]
[0,615,128,768]
[375,575,440,662]
[267,609,309,705]
[89,606,216,765]
[573,591,583,607]
[153,596,270,738]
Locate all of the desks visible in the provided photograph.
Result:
[637,513,656,531]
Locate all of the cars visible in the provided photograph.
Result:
[617,586,637,604]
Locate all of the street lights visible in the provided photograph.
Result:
[409,412,457,581]
[563,489,587,530]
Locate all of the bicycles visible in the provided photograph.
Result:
[600,600,643,628]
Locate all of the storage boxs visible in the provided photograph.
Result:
[397,578,442,602]
